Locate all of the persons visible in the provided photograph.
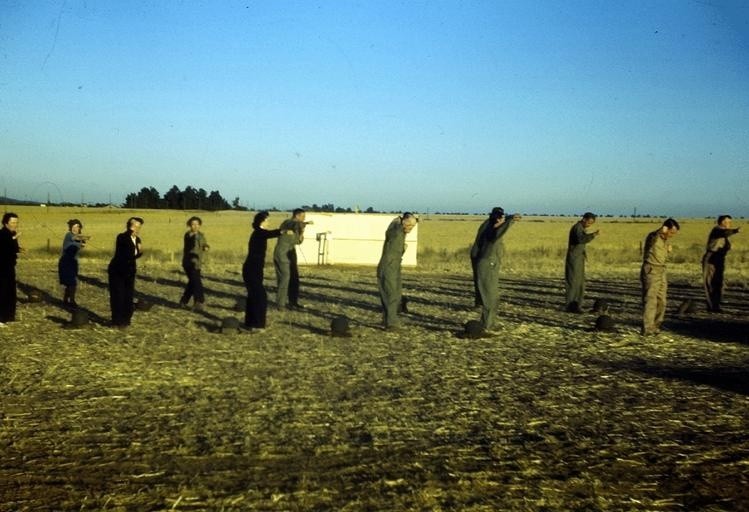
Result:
[239,209,296,334]
[177,215,212,313]
[270,206,315,309]
[375,210,418,330]
[106,215,146,327]
[469,206,506,308]
[638,217,681,337]
[0,211,28,329]
[563,211,602,314]
[473,211,523,333]
[56,218,93,309]
[699,213,743,315]
